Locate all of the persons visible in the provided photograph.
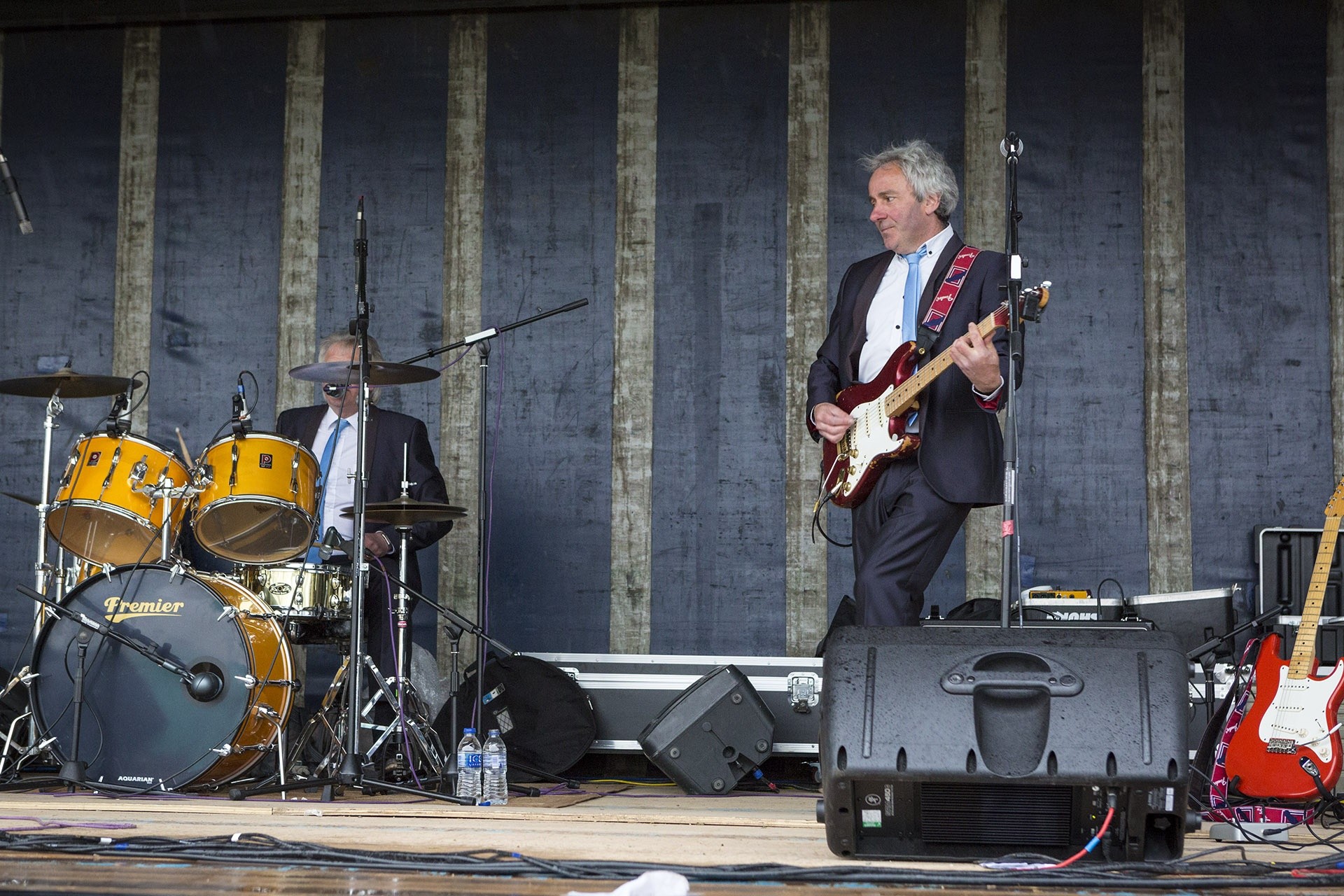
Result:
[800,141,1024,821]
[277,332,455,783]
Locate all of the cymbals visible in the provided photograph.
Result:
[0,367,143,398]
[339,496,469,526]
[288,361,442,385]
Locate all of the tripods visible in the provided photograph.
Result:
[0,229,584,809]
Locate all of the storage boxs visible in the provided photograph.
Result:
[1252,524,1344,664]
[1130,583,1241,656]
[1021,596,1121,622]
[515,651,823,756]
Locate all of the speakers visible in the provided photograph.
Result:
[815,621,1189,861]
[637,664,775,797]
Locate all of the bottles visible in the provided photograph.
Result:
[482,729,508,806]
[457,728,482,806]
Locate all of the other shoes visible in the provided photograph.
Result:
[372,746,410,776]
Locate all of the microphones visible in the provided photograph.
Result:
[323,383,344,398]
[355,201,364,296]
[0,153,32,234]
[318,526,337,560]
[237,377,253,432]
[117,379,134,432]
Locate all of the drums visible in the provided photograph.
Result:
[236,560,353,620]
[28,563,297,793]
[47,431,194,565]
[74,555,105,587]
[192,430,320,565]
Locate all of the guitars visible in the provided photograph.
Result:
[823,280,1053,510]
[1224,474,1344,802]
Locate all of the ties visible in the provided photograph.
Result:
[305,420,348,559]
[901,248,926,428]
